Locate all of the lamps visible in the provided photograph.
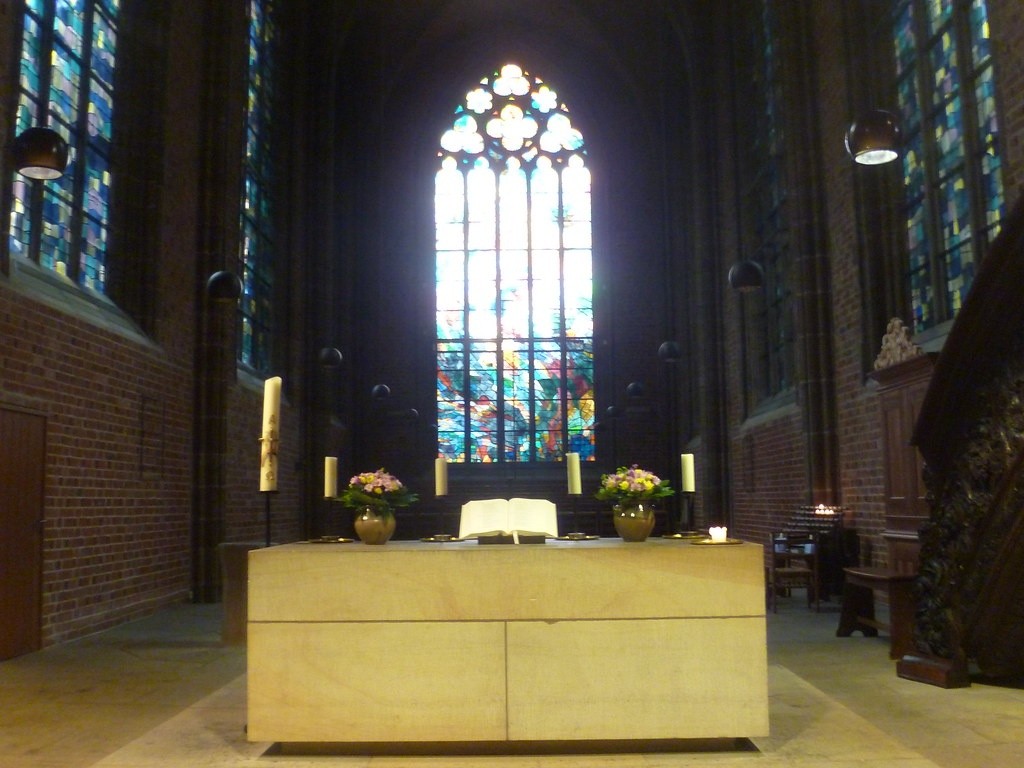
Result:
[12,125,68,180]
[627,383,647,399]
[842,89,903,165]
[658,342,681,361]
[371,385,390,402]
[606,406,619,417]
[208,271,244,301]
[317,347,343,369]
[721,260,793,317]
[404,408,420,421]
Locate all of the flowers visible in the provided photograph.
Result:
[332,468,423,518]
[595,464,677,502]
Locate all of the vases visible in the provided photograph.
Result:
[353,510,396,545]
[612,500,656,542]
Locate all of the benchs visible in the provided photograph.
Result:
[834,567,922,661]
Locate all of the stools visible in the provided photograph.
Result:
[772,566,812,611]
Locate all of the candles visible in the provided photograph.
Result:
[566,452,582,494]
[435,458,448,496]
[258,375,283,492]
[324,455,337,498]
[682,453,696,492]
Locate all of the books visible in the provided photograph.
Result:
[457,497,561,539]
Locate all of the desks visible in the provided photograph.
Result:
[247,538,772,753]
